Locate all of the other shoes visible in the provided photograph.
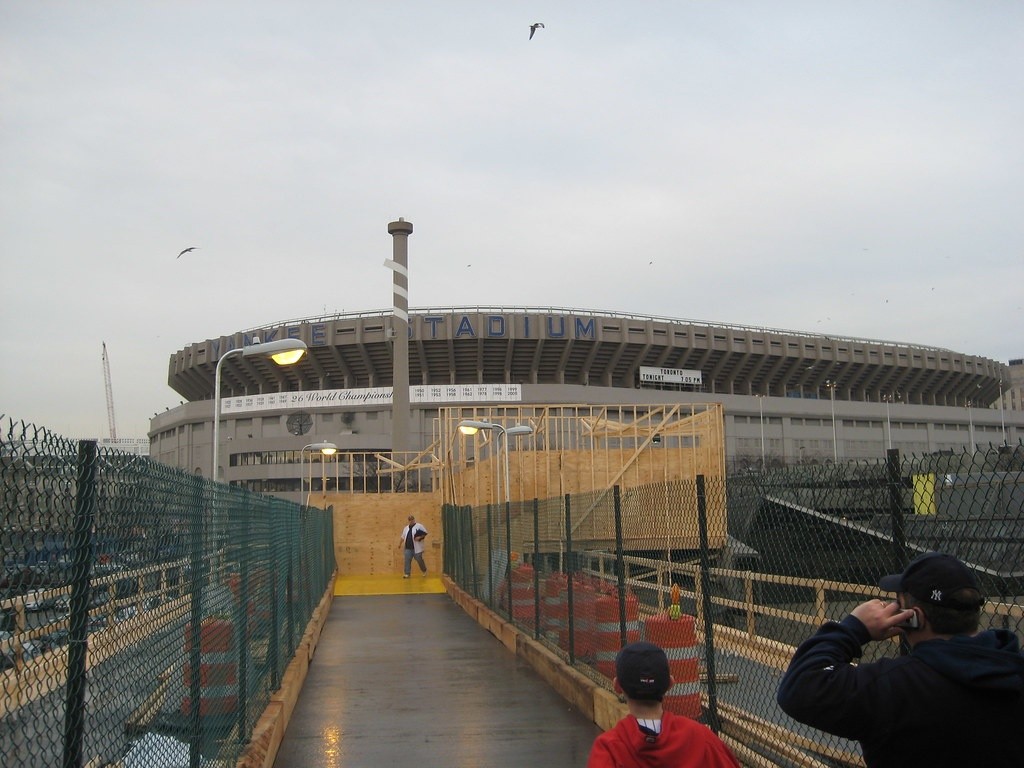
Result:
[403,574,410,578]
[423,570,427,576]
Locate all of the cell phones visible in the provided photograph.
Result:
[892,609,918,628]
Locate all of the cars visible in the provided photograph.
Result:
[0,545,204,664]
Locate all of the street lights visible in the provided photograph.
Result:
[494,425,534,568]
[299,442,337,507]
[458,420,510,503]
[207,339,308,582]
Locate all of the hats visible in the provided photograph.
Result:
[878,552,985,612]
[615,642,670,700]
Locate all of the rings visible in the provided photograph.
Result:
[880,602,885,608]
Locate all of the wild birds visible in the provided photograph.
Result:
[177,247,201,259]
[528,22,545,41]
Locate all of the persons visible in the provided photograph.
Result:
[398,515,428,578]
[587,642,741,768]
[777,552,1024,768]
